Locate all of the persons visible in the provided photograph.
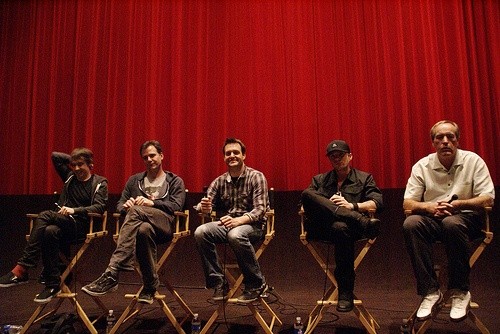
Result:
[194,137,271,302]
[300,141,385,312]
[82,139,186,304]
[402,120,495,322]
[0,148,108,303]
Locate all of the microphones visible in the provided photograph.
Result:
[448,193,459,203]
[203,186,211,217]
[54,201,76,222]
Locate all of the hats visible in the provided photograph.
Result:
[326,140,350,156]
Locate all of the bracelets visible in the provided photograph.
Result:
[352,202,359,211]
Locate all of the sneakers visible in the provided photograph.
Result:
[81,271,119,297]
[138,287,153,304]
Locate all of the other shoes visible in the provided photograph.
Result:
[450,290,471,322]
[357,216,381,240]
[0,272,28,287]
[33,285,61,303]
[337,280,353,311]
[416,289,442,320]
[213,279,229,300]
[237,283,268,301]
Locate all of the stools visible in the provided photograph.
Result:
[298,202,379,334]
[400,207,493,334]
[17,192,108,334]
[196,186,283,334]
[108,189,198,334]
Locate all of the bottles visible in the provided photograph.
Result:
[106,310,116,334]
[294,317,304,334]
[400,319,411,334]
[191,313,201,334]
[3,325,23,334]
